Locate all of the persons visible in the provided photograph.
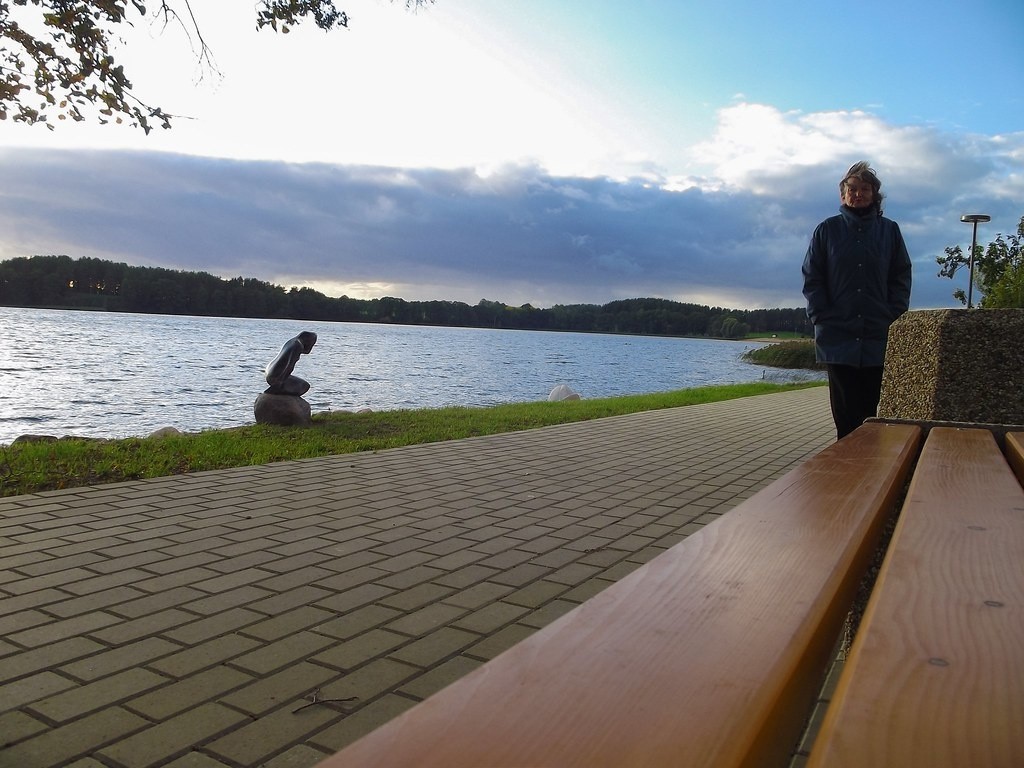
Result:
[801,161,912,441]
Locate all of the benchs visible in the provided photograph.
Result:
[314,421,1024,768]
